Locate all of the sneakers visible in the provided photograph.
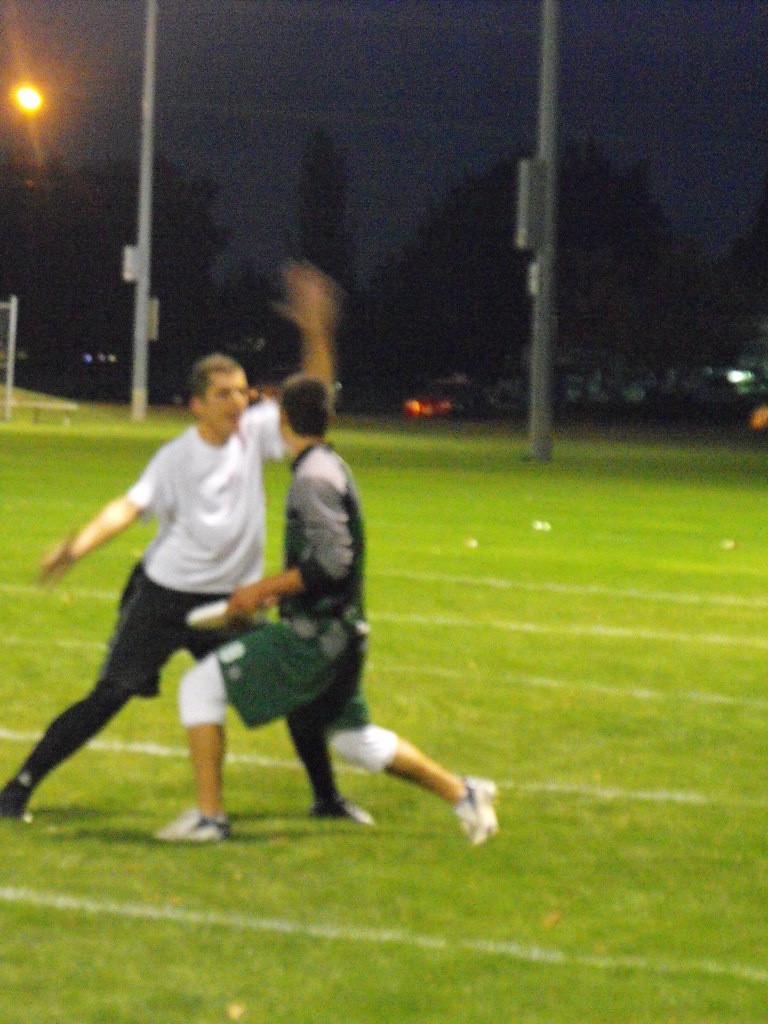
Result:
[457,776,499,846]
[155,813,230,843]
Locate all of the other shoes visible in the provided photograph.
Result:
[310,799,377,827]
[0,780,29,818]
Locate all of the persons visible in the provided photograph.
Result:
[0,264,369,823]
[147,374,497,847]
